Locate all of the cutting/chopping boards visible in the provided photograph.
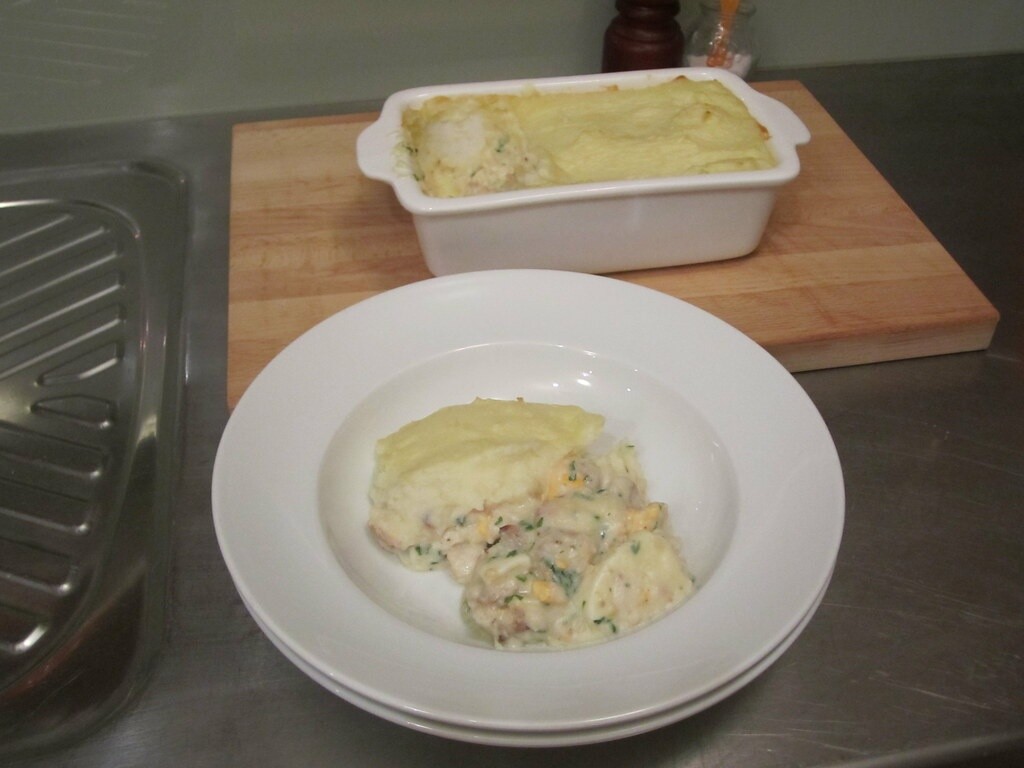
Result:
[227,79,999,416]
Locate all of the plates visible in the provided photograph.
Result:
[211,268,846,749]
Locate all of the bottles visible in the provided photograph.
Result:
[683,0,756,82]
[601,0,685,73]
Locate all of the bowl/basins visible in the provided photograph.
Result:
[356,66,810,276]
